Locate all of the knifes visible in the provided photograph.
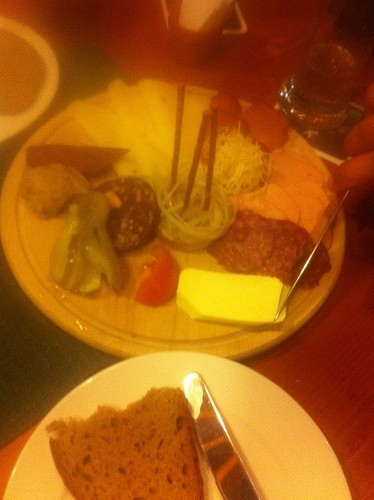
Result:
[274,186,352,324]
[181,371,261,499]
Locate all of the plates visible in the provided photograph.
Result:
[0,83,346,366]
[0,18,60,141]
[4,350,353,499]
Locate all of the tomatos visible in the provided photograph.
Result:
[134,244,178,306]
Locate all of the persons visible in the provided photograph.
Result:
[326,82,374,206]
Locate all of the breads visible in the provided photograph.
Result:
[44,387,202,500]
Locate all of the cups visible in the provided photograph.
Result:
[275,42,357,147]
[160,1,247,54]
